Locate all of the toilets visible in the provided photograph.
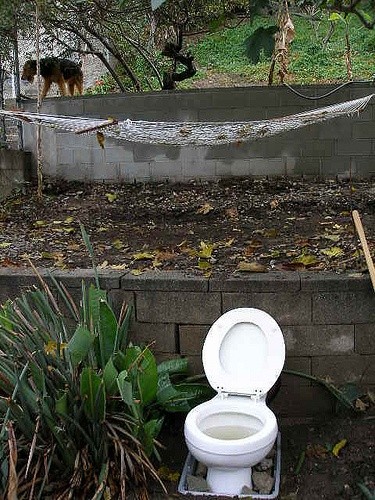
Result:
[184,307,286,495]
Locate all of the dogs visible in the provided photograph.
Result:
[20,57,84,109]
